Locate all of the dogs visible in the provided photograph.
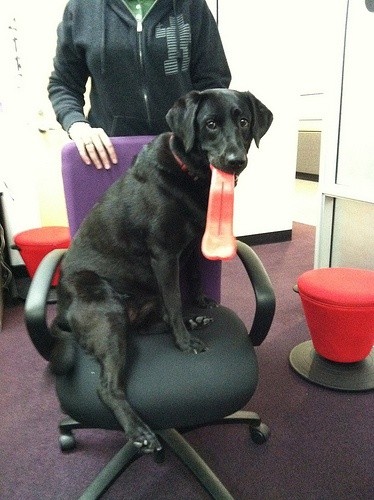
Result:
[45,86,275,454]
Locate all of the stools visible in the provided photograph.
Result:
[288,267,373,393]
[15,227,71,304]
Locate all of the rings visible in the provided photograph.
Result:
[86,143,93,145]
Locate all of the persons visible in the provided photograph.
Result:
[48,0,232,172]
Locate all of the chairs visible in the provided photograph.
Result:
[24,136,277,500]
